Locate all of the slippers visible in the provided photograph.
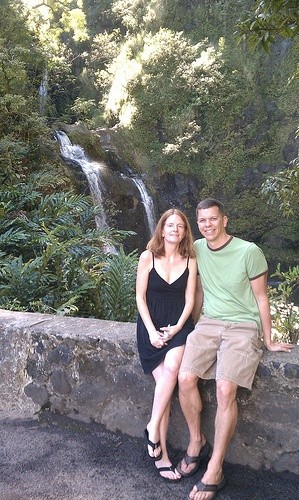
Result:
[175,442,212,476]
[143,428,162,462]
[153,459,181,483]
[187,473,226,500]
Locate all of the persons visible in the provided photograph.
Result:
[177,198,295,500]
[136,208,197,482]
